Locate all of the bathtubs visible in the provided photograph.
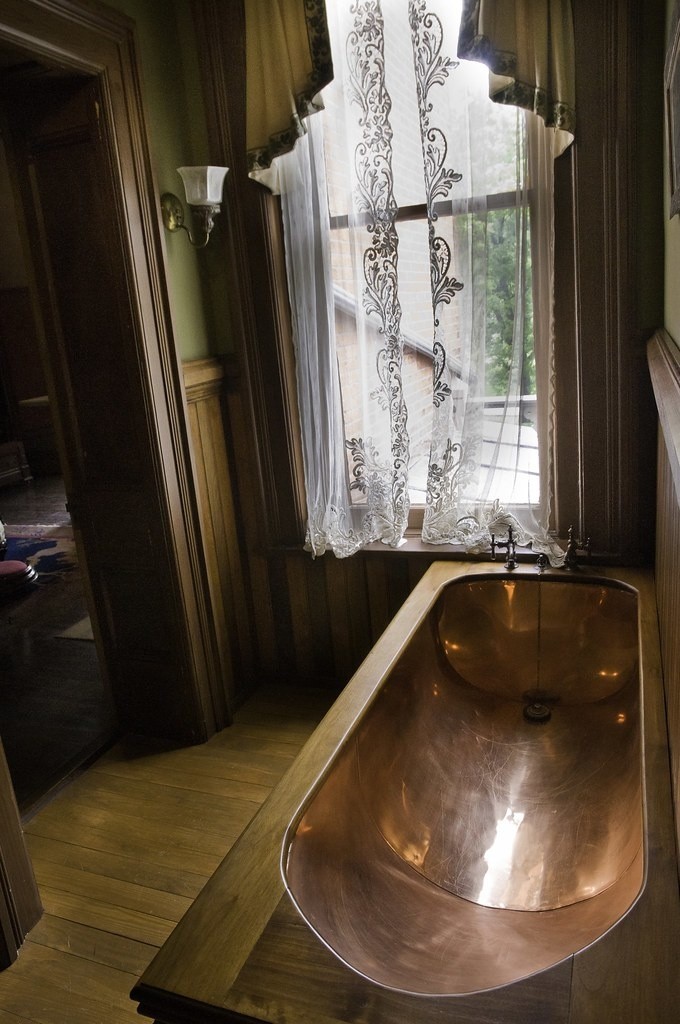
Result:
[269,567,657,1004]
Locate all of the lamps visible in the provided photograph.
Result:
[160,164,229,247]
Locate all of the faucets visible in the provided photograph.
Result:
[562,524,593,572]
[490,523,519,570]
[536,553,548,572]
